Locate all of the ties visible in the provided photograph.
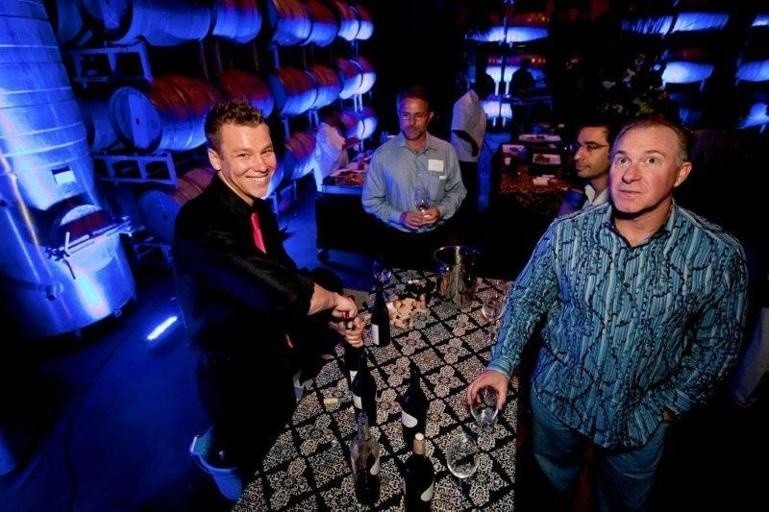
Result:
[249,211,266,254]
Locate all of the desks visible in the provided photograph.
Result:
[222,268,535,509]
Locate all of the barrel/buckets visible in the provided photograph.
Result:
[434,245,479,309]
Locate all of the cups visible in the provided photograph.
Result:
[479,291,503,349]
[445,431,481,498]
[414,196,436,230]
[469,383,502,439]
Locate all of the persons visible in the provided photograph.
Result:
[557,115,621,220]
[509,58,535,143]
[312,105,361,201]
[171,94,366,494]
[466,114,748,510]
[451,72,496,227]
[361,86,467,272]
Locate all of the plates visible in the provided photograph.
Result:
[531,153,561,166]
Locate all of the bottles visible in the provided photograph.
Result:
[349,409,381,507]
[400,366,429,450]
[402,431,437,511]
[433,244,483,310]
[342,316,368,392]
[351,353,377,429]
[370,279,391,348]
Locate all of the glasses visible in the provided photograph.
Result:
[575,142,610,151]
